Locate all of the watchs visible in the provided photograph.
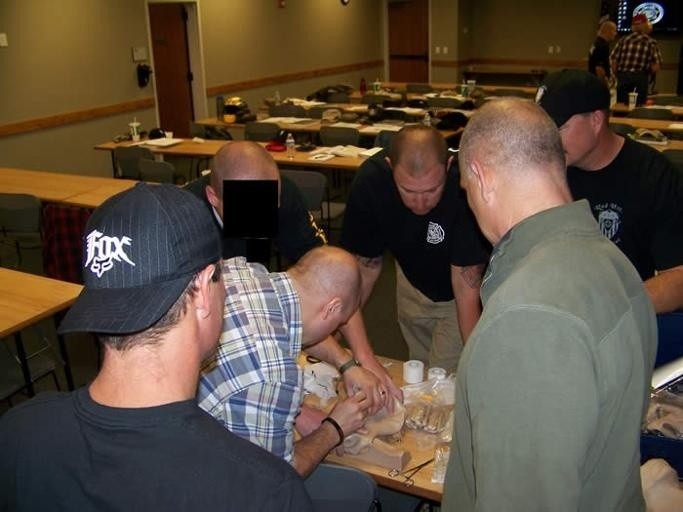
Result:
[340,359,362,373]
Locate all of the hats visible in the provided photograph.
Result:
[533,67,611,128]
[631,13,648,25]
[55,180,222,337]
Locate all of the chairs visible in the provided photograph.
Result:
[277,171,346,245]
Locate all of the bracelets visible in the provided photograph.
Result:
[320,417,344,446]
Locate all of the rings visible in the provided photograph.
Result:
[379,391,385,396]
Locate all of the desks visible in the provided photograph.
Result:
[290,348,456,511]
[256,83,538,121]
[93,113,465,188]
[609,93,683,164]
[2,167,139,409]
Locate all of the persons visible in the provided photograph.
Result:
[610,13,663,104]
[441,94,660,511]
[341,124,480,375]
[535,68,683,371]
[195,244,372,479]
[183,141,403,418]
[589,21,616,77]
[0,180,320,511]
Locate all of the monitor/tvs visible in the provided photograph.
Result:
[600,0,683,40]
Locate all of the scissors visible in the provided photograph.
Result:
[387,455,436,488]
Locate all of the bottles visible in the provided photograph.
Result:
[256,105,271,121]
[359,77,367,97]
[216,95,226,121]
[274,89,282,107]
[286,133,295,159]
[423,112,431,126]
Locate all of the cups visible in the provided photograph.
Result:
[129,122,142,142]
[164,131,173,144]
[372,83,381,94]
[628,92,639,109]
[401,358,447,385]
[461,85,468,97]
